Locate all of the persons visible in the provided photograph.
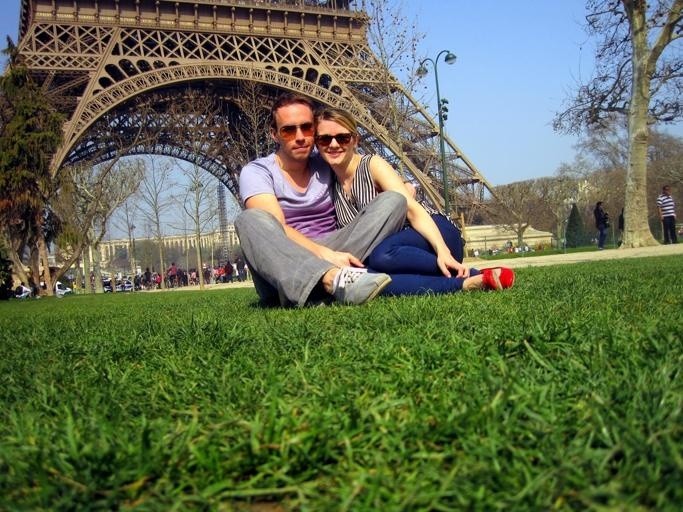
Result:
[312,100,514,293]
[593,200,609,249]
[66,258,244,294]
[230,95,406,307]
[655,185,677,244]
[470,240,543,257]
[617,208,624,246]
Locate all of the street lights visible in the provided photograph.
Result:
[131,224,137,275]
[416,50,457,218]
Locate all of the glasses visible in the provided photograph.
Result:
[314,133,354,146]
[279,123,314,142]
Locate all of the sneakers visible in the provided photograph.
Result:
[481,270,503,291]
[332,265,393,307]
[479,266,515,290]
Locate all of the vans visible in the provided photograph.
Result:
[39,281,72,297]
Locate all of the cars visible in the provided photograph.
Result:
[72,271,135,293]
